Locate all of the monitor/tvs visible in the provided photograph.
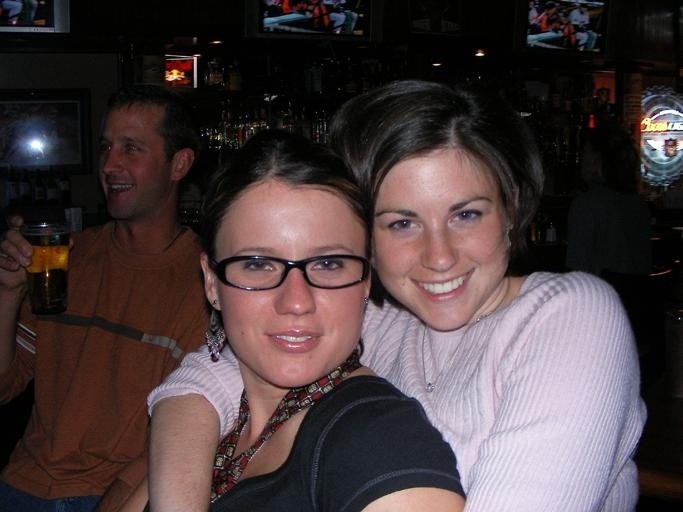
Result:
[0,0,71,34]
[516,0,611,60]
[159,56,198,88]
[243,0,385,43]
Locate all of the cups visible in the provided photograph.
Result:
[19,222,72,316]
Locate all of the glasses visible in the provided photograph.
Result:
[207,251,370,292]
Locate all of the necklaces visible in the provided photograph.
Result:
[419,312,492,393]
[210,347,362,502]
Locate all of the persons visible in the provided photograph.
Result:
[121,129,466,512]
[1,83,214,512]
[563,123,650,274]
[146,79,651,512]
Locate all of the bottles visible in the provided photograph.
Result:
[7,163,71,209]
[545,220,558,243]
[656,186,673,209]
[311,111,329,145]
[200,103,267,151]
